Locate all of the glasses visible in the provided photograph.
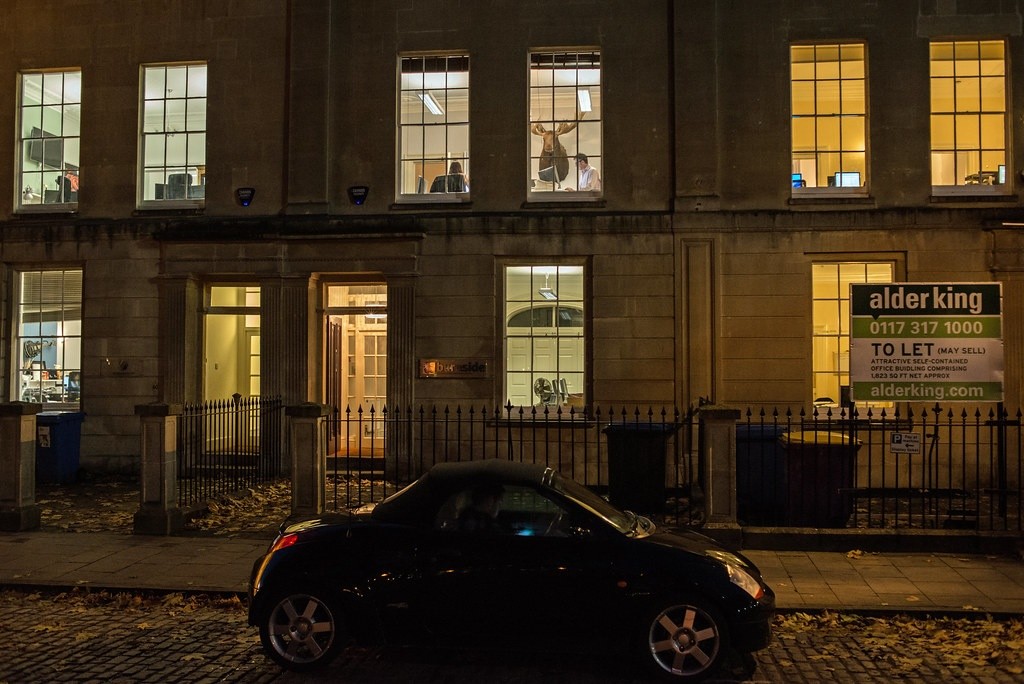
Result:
[575,160,581,164]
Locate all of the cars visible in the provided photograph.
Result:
[246,459,775,683]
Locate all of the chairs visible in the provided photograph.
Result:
[34,394,48,402]
[435,175,464,192]
[52,175,71,203]
[169,173,193,199]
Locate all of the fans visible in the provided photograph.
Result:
[533,376,553,406]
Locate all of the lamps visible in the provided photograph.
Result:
[538,273,558,300]
[23,185,46,201]
[578,88,592,111]
[416,90,445,116]
[365,286,386,318]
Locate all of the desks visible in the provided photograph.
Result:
[28,379,62,389]
[48,392,69,401]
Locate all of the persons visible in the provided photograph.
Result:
[449,162,469,188]
[462,483,513,535]
[70,374,79,387]
[566,153,601,191]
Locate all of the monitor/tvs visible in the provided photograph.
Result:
[29,127,62,170]
[538,165,561,189]
[417,176,427,194]
[792,174,802,187]
[835,172,860,187]
[998,165,1006,184]
[827,177,836,187]
[552,378,568,395]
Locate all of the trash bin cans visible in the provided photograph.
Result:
[34,412,85,494]
[737,426,787,527]
[602,422,674,527]
[780,430,862,529]
